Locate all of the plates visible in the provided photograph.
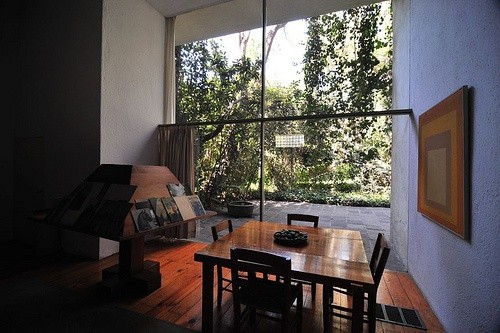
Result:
[273,232,308,242]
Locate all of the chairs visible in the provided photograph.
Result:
[211,213,319,333]
[323,232,390,333]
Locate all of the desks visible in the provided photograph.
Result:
[194,219,375,333]
